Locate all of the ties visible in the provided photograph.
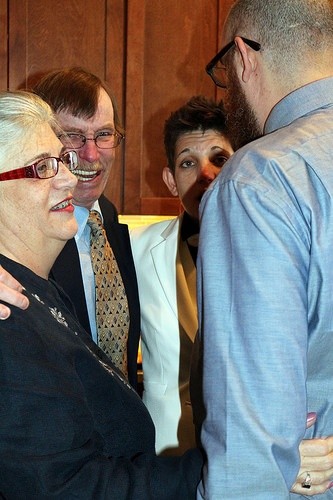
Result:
[86,210,130,385]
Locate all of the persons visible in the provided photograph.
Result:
[0,67,142,391]
[131,96,236,456]
[194,0,333,500]
[0,89,333,500]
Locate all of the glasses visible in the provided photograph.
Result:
[58,127,126,150]
[0,150,79,182]
[205,36,262,88]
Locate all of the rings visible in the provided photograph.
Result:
[301,473,313,489]
